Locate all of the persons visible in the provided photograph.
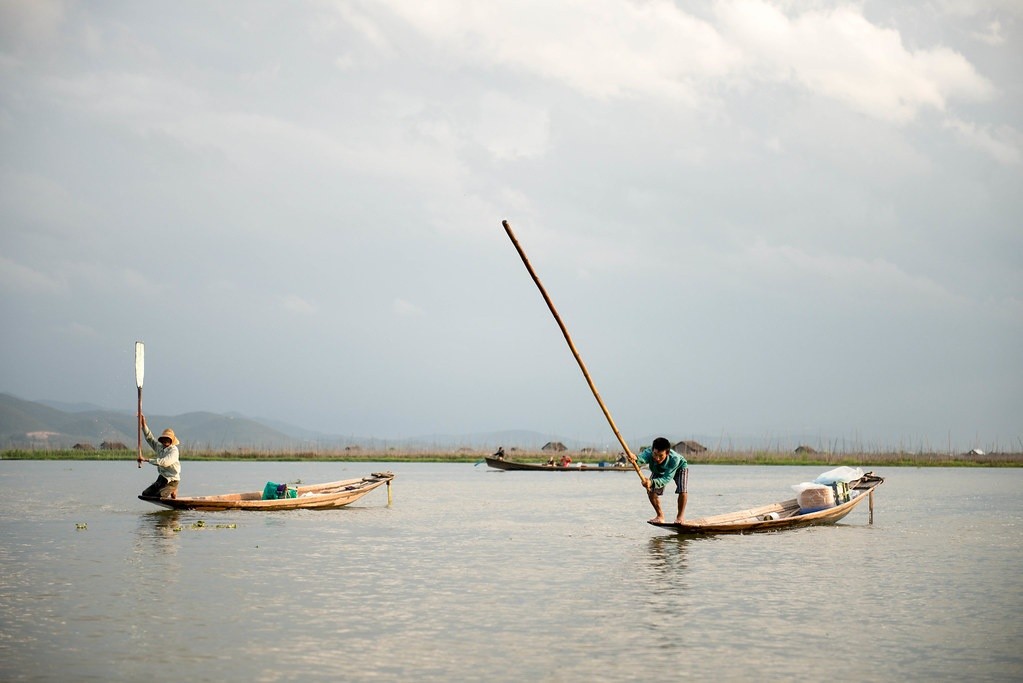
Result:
[617,453,626,467]
[136,413,181,498]
[626,437,688,523]
[549,455,572,466]
[494,448,504,461]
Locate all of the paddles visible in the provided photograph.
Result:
[500,217,649,490]
[474,453,498,468]
[132,340,146,469]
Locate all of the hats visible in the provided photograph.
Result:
[158,429,174,443]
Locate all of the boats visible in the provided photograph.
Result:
[135,466,401,511]
[646,463,886,537]
[484,453,640,471]
